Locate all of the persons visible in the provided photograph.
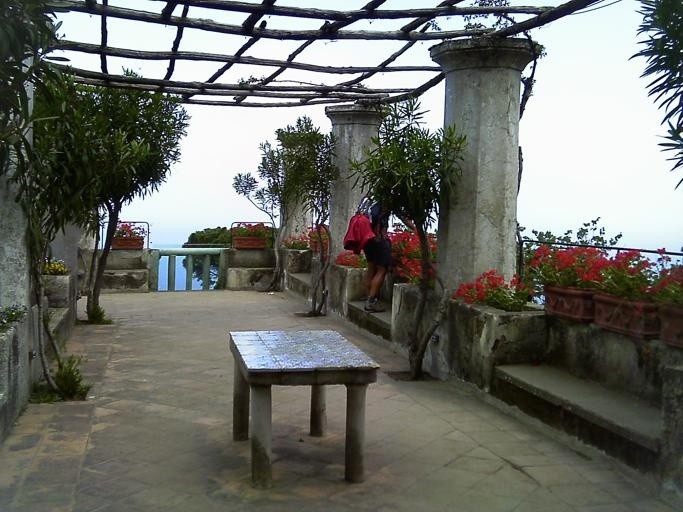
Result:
[354,191,418,313]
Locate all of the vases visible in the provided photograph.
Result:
[542,284,595,322]
[593,289,660,341]
[110,237,144,251]
[309,239,327,253]
[234,235,264,250]
[659,303,683,349]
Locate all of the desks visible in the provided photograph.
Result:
[226,329,380,491]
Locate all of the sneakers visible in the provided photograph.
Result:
[364,298,386,313]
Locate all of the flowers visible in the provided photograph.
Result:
[593,250,659,300]
[113,224,147,238]
[655,247,683,308]
[527,246,607,289]
[304,223,328,241]
[232,222,269,240]
